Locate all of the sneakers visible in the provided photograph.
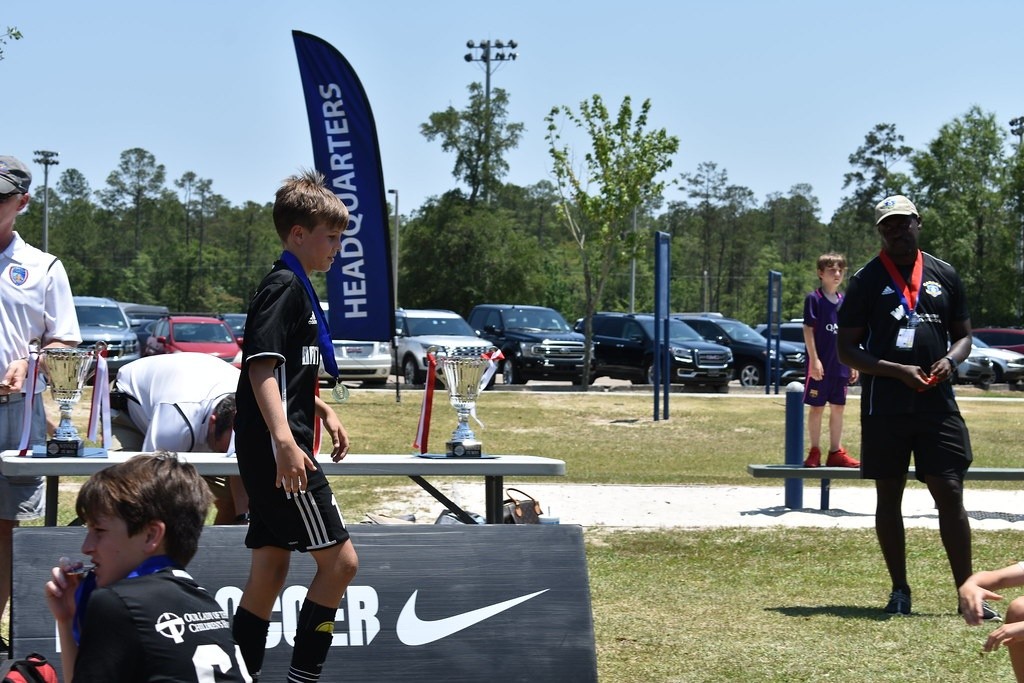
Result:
[957,599,1002,622]
[826,448,861,468]
[884,585,912,617]
[804,447,821,468]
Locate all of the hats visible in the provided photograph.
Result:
[874,195,919,226]
[0,155,32,194]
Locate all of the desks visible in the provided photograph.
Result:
[0,449,566,526]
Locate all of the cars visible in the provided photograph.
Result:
[971,337,1024,383]
[943,340,995,385]
[317,301,393,386]
[753,319,805,353]
[971,327,1024,355]
[212,312,248,350]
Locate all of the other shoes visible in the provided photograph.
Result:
[0,636,10,655]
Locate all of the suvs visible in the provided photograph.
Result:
[670,312,811,387]
[393,308,500,391]
[466,305,594,386]
[572,312,734,389]
[68,295,139,373]
[144,312,242,370]
[116,302,170,341]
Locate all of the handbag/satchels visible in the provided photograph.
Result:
[503,488,543,524]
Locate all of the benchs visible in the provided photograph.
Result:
[11,524,598,683]
[747,464,1024,509]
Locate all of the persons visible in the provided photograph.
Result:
[108,350,250,525]
[0,153,83,654]
[228,178,358,683]
[44,453,256,683]
[802,252,860,468]
[958,562,1024,683]
[838,194,1003,625]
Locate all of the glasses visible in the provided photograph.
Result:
[0,192,27,203]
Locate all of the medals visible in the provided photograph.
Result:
[67,564,96,577]
[908,313,918,326]
[331,383,350,403]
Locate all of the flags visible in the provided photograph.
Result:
[291,28,396,343]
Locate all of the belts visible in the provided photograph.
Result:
[0,393,26,404]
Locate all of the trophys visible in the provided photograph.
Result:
[29,338,107,458]
[425,345,498,459]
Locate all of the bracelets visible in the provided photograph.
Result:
[22,357,29,378]
[945,356,957,373]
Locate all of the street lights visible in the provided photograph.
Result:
[32,149,61,254]
[385,188,398,312]
[462,36,519,209]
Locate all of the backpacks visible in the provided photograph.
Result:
[0,653,59,683]
